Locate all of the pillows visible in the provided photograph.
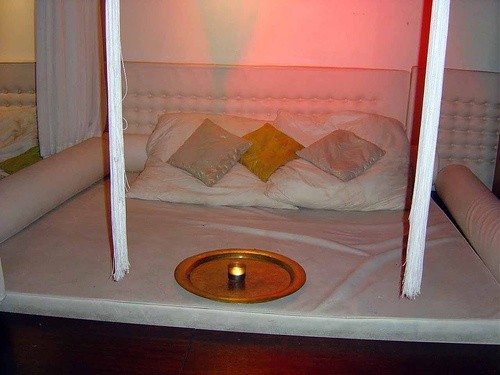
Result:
[236,123,306,183]
[127,113,300,210]
[166,119,251,187]
[264,109,412,211]
[295,129,387,181]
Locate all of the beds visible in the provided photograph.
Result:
[1,62,500,344]
